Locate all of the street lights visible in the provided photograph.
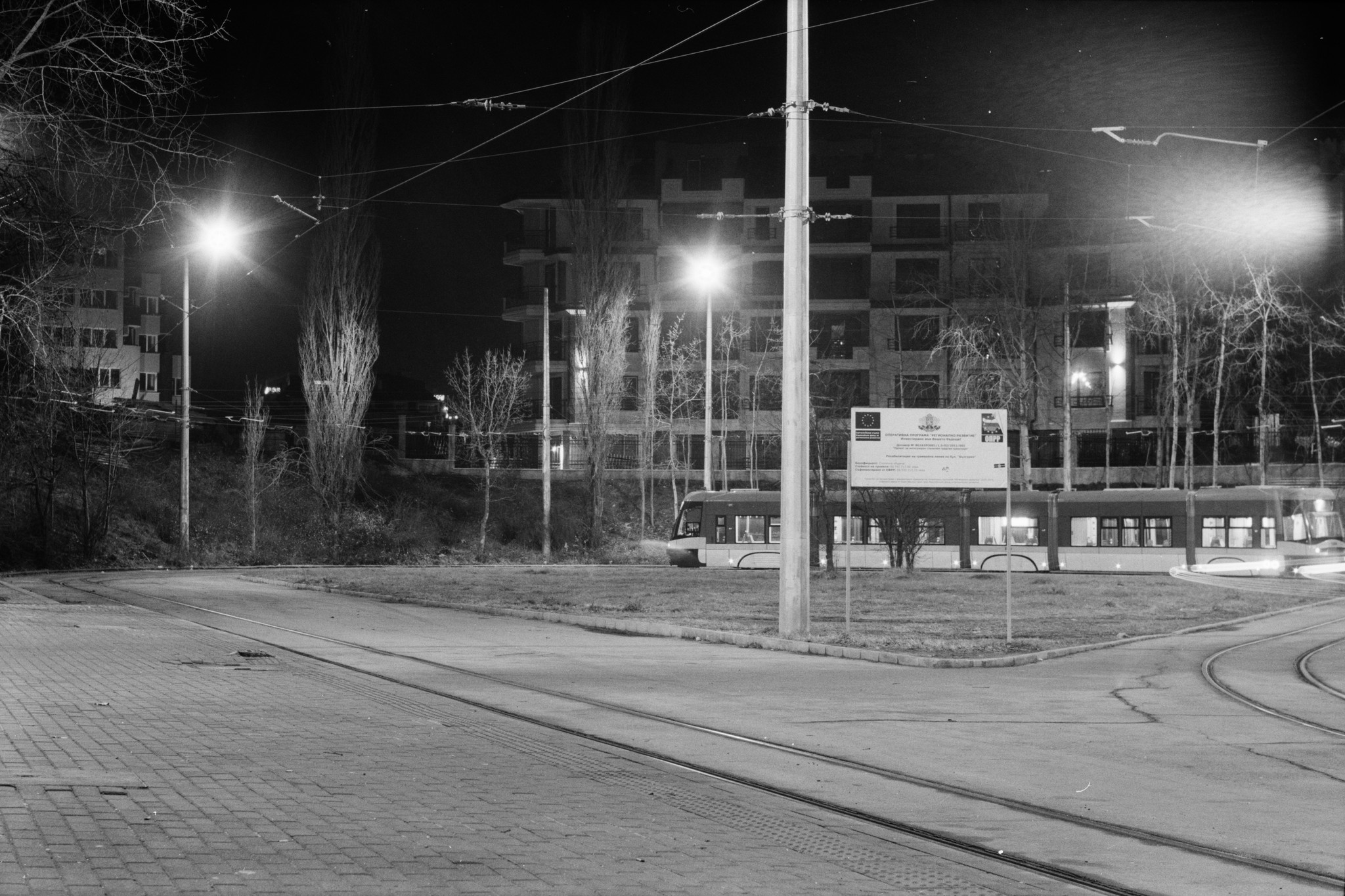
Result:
[179,228,234,551]
[689,254,725,490]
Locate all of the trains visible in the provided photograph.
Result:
[666,485,1345,574]
